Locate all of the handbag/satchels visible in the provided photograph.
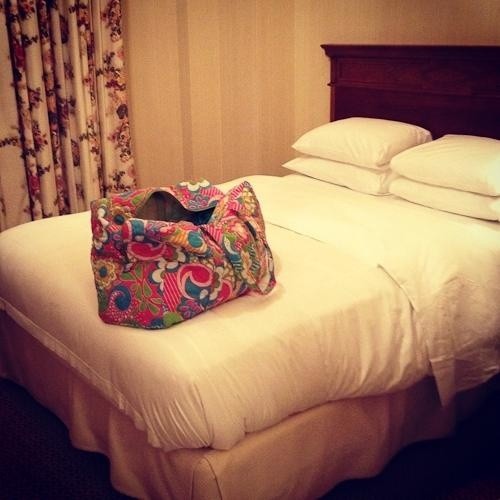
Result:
[90,177,276,329]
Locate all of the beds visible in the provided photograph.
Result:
[1,39,500,497]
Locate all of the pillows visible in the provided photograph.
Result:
[279,116,500,222]
